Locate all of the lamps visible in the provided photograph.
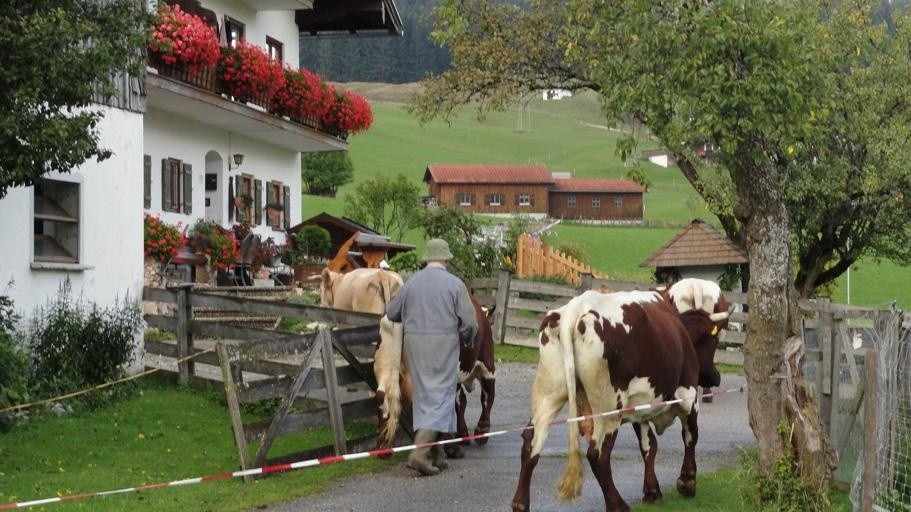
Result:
[227,151,243,172]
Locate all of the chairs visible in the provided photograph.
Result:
[228,229,262,287]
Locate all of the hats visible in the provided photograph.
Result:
[423,238,454,261]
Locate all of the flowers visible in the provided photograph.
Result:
[258,234,291,268]
[144,212,244,285]
[148,0,376,138]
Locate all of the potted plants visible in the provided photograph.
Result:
[233,191,284,220]
[292,222,332,287]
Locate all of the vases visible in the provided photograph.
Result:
[253,264,268,280]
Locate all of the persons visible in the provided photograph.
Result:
[386,237,480,474]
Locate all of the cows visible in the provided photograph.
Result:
[371,286,498,463]
[314,265,402,314]
[507,288,738,512]
[648,276,730,404]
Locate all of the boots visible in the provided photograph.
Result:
[405,428,452,478]
